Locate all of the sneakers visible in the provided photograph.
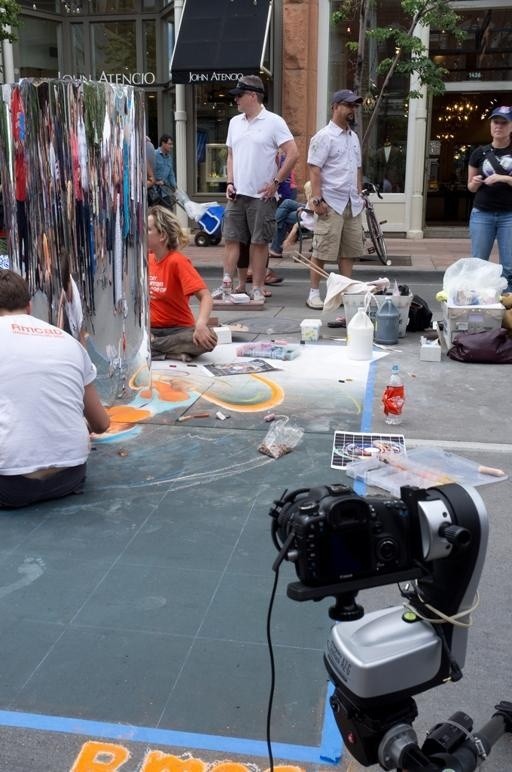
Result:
[211,286,247,301]
[247,270,283,301]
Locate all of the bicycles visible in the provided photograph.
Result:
[359,179,391,266]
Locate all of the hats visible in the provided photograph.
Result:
[331,89,363,104]
[488,106,512,122]
[228,81,264,97]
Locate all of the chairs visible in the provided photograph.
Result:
[295,208,314,261]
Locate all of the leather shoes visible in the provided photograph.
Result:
[306,296,324,310]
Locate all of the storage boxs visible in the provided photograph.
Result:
[441,301,505,349]
[342,288,412,337]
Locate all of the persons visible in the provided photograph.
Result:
[212,75,364,310]
[146,137,155,187]
[147,205,218,363]
[52,261,85,343]
[467,106,512,293]
[0,269,110,510]
[372,135,401,193]
[149,135,176,208]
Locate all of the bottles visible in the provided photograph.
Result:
[222,273,232,302]
[384,366,405,425]
[254,288,261,302]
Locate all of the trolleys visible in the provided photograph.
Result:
[155,183,226,250]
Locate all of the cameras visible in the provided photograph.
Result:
[272,484,423,621]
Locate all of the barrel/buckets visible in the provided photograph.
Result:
[373,297,401,345]
